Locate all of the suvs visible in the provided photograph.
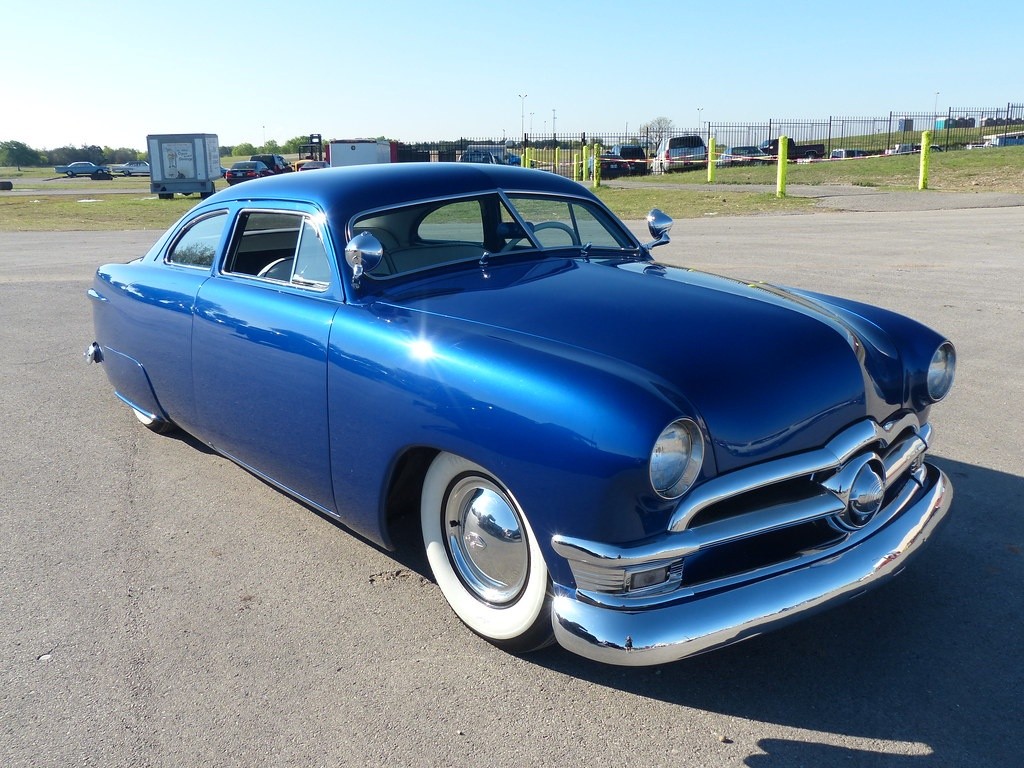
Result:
[606,144,647,175]
[647,135,709,175]
[250,154,293,174]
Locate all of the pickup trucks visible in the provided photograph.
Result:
[759,136,825,162]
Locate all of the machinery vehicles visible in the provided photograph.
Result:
[294,133,323,172]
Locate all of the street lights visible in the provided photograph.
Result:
[697,107,703,135]
[935,91,940,113]
[518,94,529,145]
[529,112,535,134]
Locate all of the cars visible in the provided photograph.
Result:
[579,153,630,181]
[830,148,870,160]
[54,161,108,178]
[715,146,776,169]
[224,161,276,186]
[479,513,522,541]
[111,161,150,176]
[220,165,229,179]
[293,158,329,172]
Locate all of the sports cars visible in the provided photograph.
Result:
[83,159,960,668]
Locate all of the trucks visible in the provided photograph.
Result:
[467,144,535,167]
[457,150,496,164]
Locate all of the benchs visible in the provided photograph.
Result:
[232,227,494,289]
